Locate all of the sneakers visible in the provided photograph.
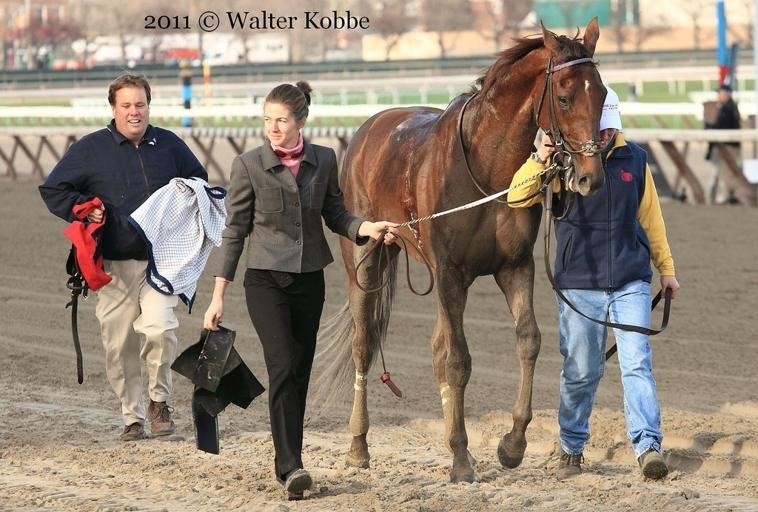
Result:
[557,450,585,481]
[120,422,146,441]
[285,469,312,500]
[638,448,669,482]
[149,401,175,436]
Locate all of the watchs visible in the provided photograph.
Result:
[529,151,547,165]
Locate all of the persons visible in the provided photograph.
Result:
[37,75,210,444]
[702,85,743,203]
[204,79,401,501]
[504,87,679,481]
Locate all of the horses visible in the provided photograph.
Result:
[309,14,609,485]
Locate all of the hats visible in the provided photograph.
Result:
[600,85,624,132]
